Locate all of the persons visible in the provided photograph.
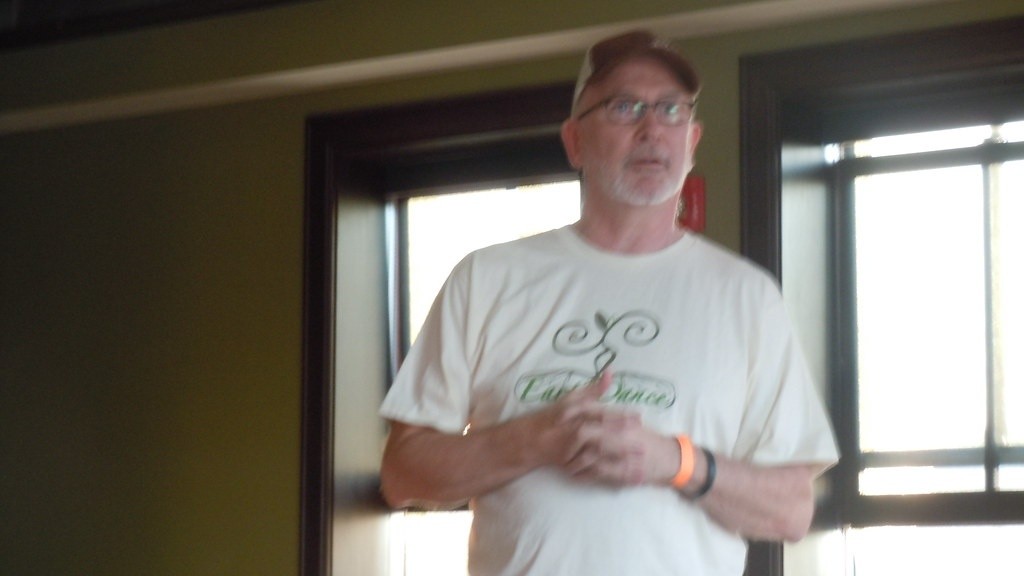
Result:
[379,26,841,574]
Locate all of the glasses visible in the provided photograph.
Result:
[573,95,700,127]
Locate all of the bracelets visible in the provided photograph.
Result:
[668,434,697,487]
[685,449,717,501]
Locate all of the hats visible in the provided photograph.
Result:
[571,30,701,117]
